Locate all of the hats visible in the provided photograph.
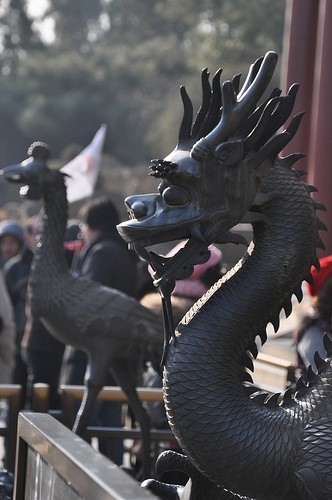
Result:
[0,223,24,248]
[166,239,222,280]
[307,254,332,297]
[63,219,82,241]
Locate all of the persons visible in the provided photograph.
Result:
[0,219,332,386]
[58,198,134,465]
